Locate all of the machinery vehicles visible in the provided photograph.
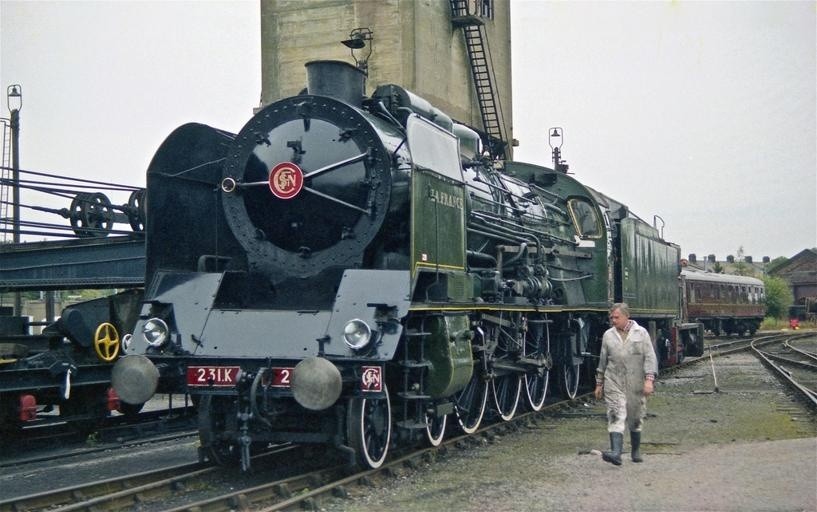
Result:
[0,284,143,445]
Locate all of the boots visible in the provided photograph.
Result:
[630,431,643,463]
[601,431,624,465]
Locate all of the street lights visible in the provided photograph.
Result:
[5,84,25,317]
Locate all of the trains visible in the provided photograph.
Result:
[678,264,767,340]
[105,19,711,478]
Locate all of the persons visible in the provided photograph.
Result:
[595,302,658,465]
